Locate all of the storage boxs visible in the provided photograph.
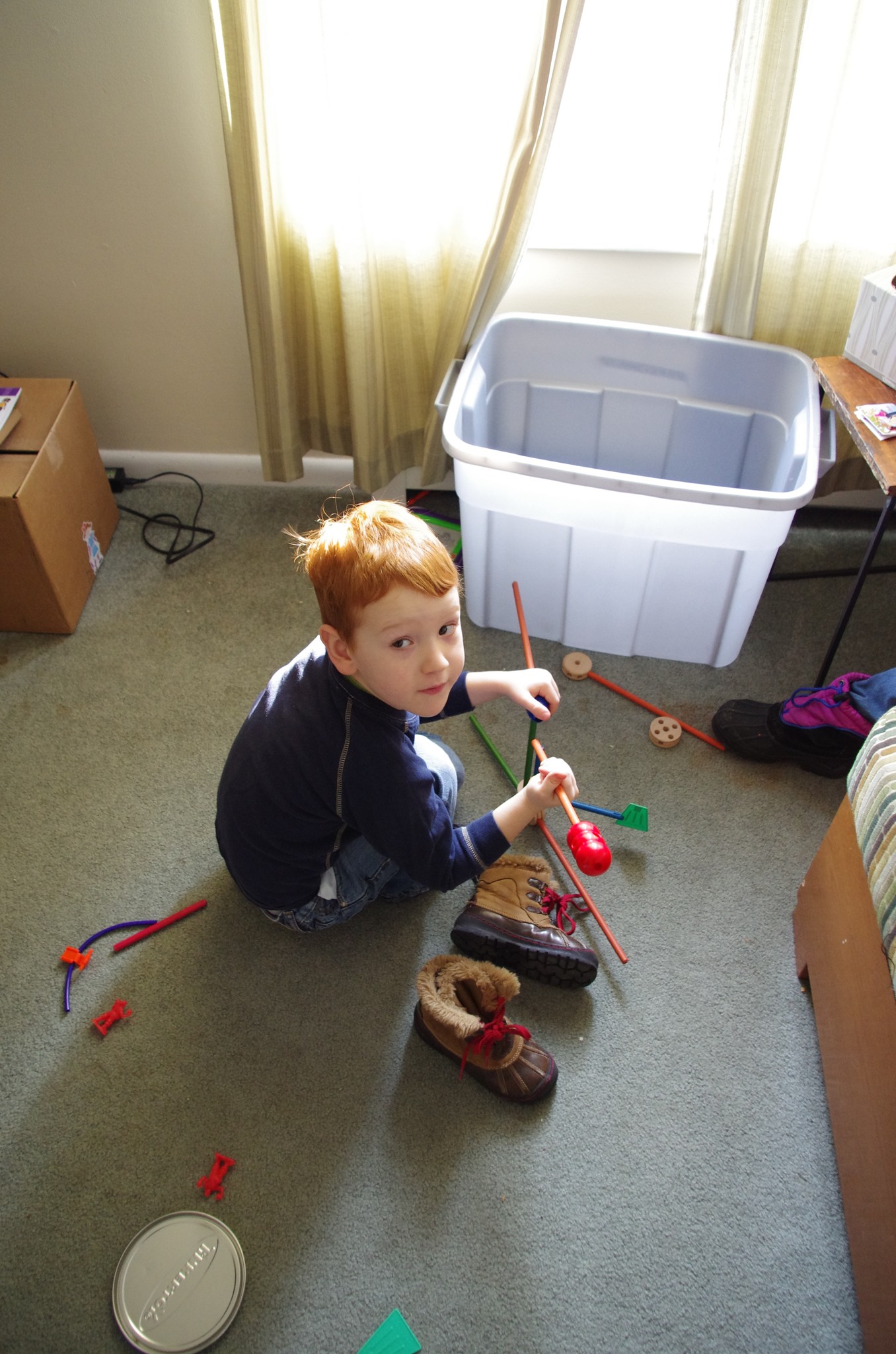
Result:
[0,375,123,640]
[840,263,896,395]
[435,313,837,670]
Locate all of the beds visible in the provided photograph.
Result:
[795,702,896,1354]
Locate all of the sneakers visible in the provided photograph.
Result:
[412,955,558,1098]
[446,854,598,992]
[711,671,876,779]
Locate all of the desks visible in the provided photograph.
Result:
[808,351,896,693]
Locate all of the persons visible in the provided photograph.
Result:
[215,495,581,932]
